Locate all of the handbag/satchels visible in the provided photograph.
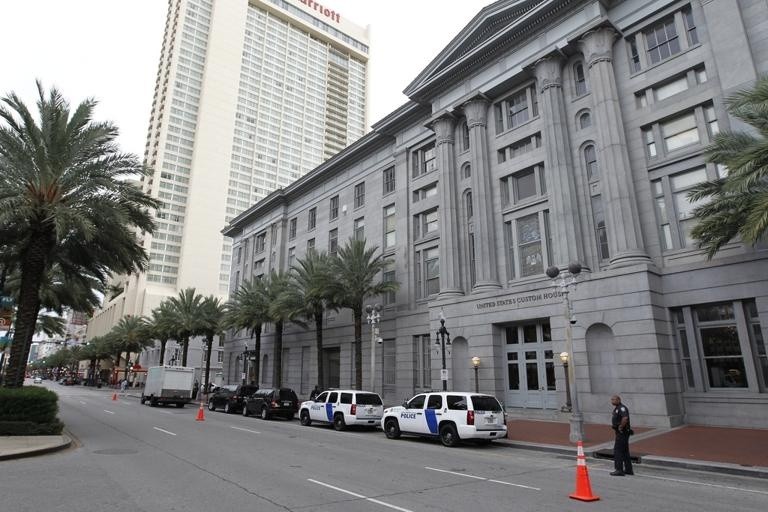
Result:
[630,430,634,435]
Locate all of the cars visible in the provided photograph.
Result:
[33,377,42,383]
[198,382,221,393]
[62,375,75,385]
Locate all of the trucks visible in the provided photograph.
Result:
[139,364,197,410]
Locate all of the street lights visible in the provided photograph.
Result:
[237,341,252,386]
[472,356,481,393]
[558,348,573,414]
[363,301,384,395]
[544,262,588,443]
[435,317,453,392]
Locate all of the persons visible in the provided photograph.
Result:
[310,385,321,401]
[121,379,128,393]
[610,394,633,476]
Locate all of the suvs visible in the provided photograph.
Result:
[207,382,260,414]
[377,391,508,449]
[240,386,302,422]
[296,387,386,433]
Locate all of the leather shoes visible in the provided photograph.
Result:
[610,471,624,476]
[625,470,634,475]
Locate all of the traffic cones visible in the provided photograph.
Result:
[193,401,205,421]
[567,440,601,503]
[112,393,117,401]
